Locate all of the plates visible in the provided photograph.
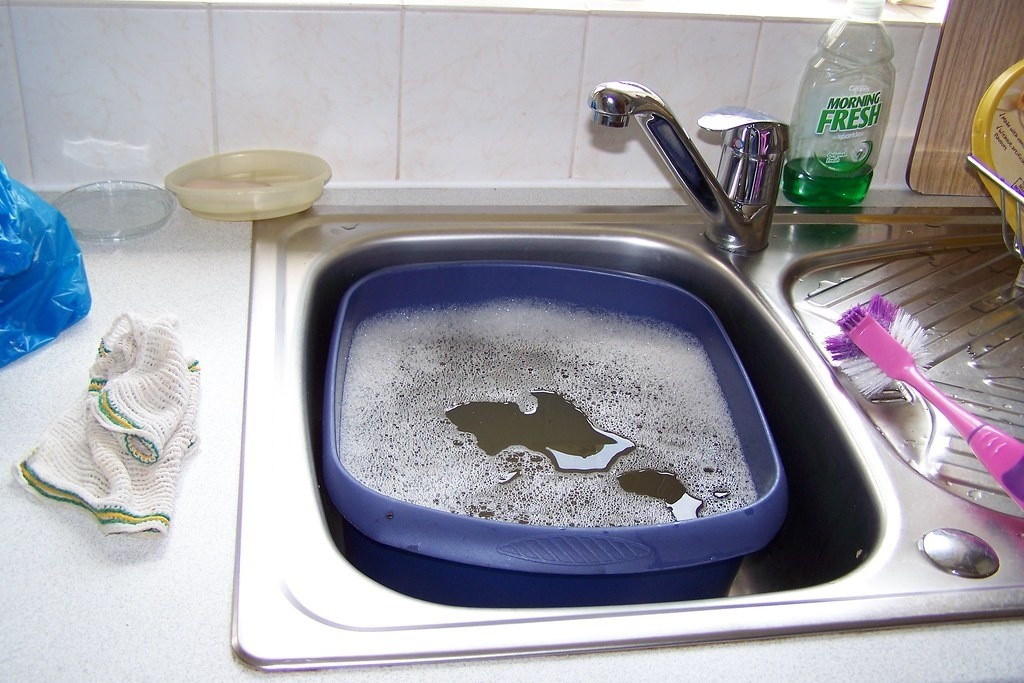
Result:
[168,150,330,219]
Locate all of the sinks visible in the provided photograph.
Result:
[229,210,905,671]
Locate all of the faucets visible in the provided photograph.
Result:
[588,80,788,266]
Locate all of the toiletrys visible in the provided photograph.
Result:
[781,0,894,204]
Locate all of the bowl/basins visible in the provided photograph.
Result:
[326,262,785,609]
